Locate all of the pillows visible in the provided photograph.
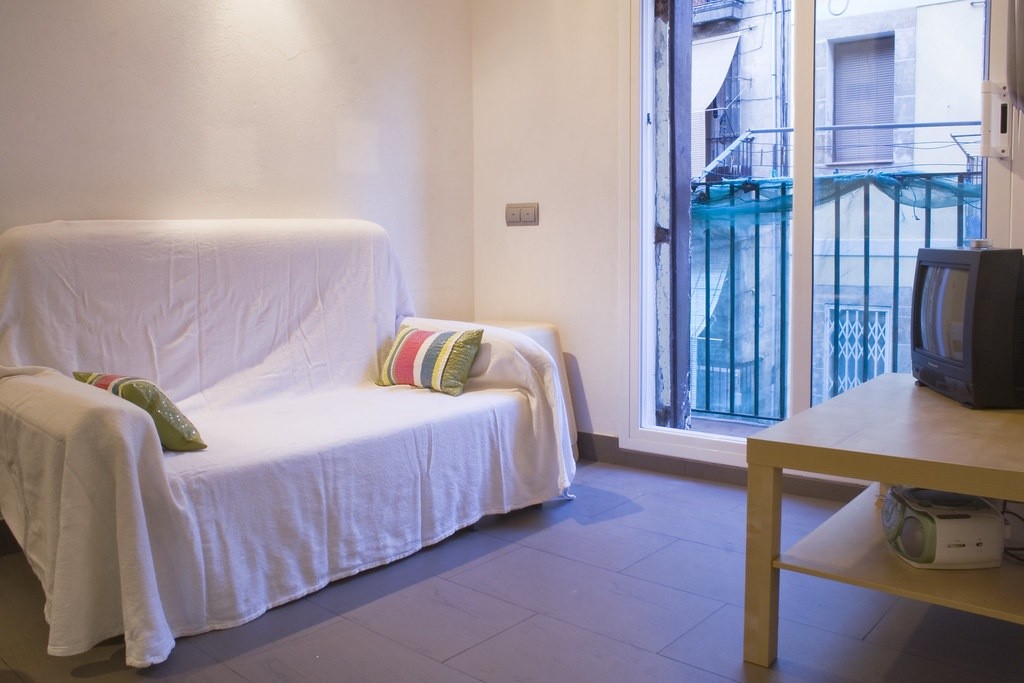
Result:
[72,371,208,451]
[375,324,484,397]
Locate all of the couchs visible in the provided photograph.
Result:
[0,218,575,668]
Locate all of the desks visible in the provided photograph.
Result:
[744,372,1024,668]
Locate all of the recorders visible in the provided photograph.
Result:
[881,485,1005,569]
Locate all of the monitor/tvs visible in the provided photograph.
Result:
[911,248,1024,410]
